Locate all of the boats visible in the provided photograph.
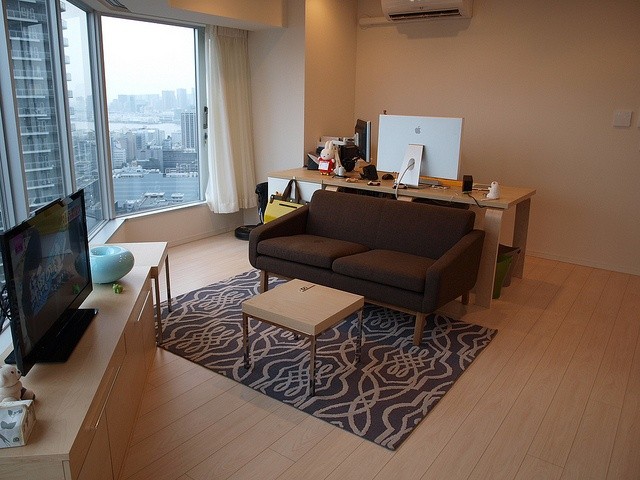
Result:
[124,192,188,210]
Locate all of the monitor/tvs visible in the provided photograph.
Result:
[353,118,371,163]
[376,114,464,189]
[1,189,99,379]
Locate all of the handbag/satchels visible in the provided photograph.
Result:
[263,179,307,225]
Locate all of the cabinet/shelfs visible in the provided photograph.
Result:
[0,265,159,480]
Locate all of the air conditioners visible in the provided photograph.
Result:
[382,0,473,23]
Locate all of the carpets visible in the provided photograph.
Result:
[152,268,499,451]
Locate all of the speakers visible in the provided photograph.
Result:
[462,175,473,192]
[363,165,378,180]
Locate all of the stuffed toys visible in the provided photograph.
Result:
[112,283,123,294]
[317,140,335,174]
[487,181,501,199]
[0,363,36,402]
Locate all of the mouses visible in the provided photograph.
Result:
[382,174,394,180]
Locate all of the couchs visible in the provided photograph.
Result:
[249,190,485,348]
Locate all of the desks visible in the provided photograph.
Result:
[90,241,172,345]
[266,167,537,310]
